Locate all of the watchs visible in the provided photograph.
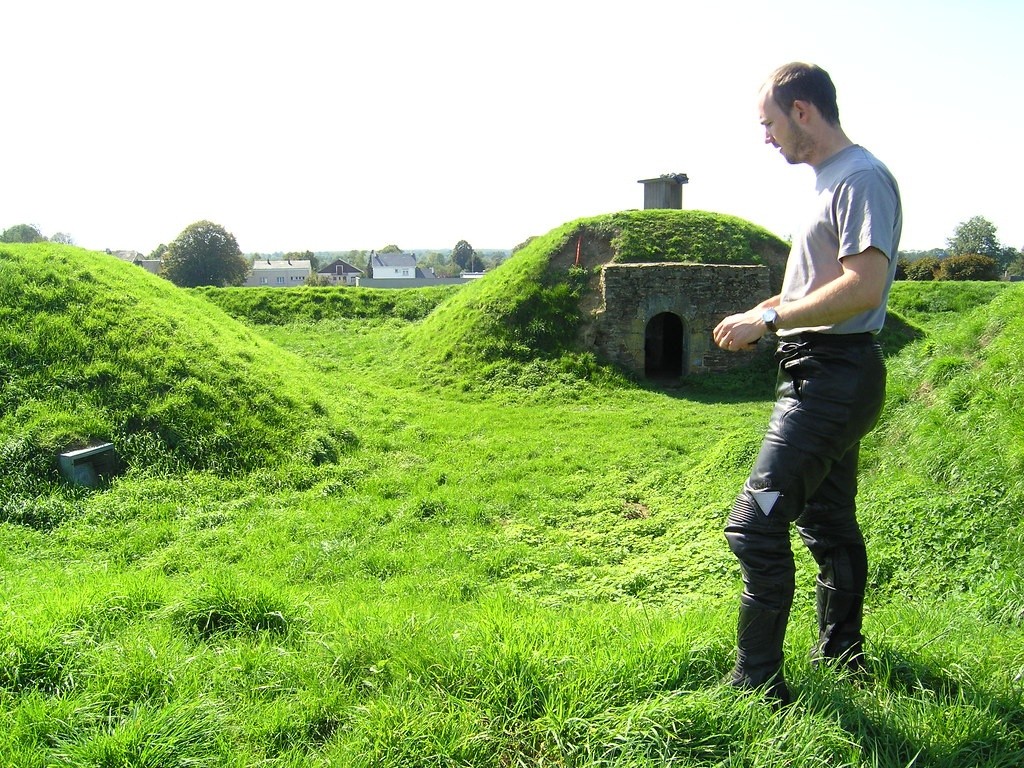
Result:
[762,306,779,332]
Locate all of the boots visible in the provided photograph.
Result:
[716,600,789,692]
[810,572,865,668]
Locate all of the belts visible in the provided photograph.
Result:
[782,331,877,349]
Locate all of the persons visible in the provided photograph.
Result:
[712,61,903,716]
